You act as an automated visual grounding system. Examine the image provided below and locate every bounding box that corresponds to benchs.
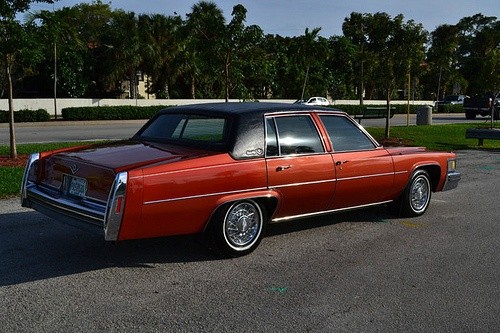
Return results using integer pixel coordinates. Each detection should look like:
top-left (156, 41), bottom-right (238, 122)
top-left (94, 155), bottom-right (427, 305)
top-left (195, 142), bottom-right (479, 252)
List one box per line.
top-left (465, 126), bottom-right (500, 147)
top-left (354, 106), bottom-right (397, 125)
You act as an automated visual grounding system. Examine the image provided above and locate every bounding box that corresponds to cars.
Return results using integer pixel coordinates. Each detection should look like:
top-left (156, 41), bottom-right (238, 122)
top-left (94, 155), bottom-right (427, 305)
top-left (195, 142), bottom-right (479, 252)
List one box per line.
top-left (293, 96), bottom-right (330, 106)
top-left (435, 94), bottom-right (471, 106)
top-left (20, 102), bottom-right (461, 257)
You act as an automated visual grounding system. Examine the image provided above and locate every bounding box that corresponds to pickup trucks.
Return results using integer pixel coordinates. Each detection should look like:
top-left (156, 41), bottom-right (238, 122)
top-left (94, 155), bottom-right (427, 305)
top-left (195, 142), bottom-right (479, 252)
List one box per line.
top-left (463, 89), bottom-right (500, 120)
